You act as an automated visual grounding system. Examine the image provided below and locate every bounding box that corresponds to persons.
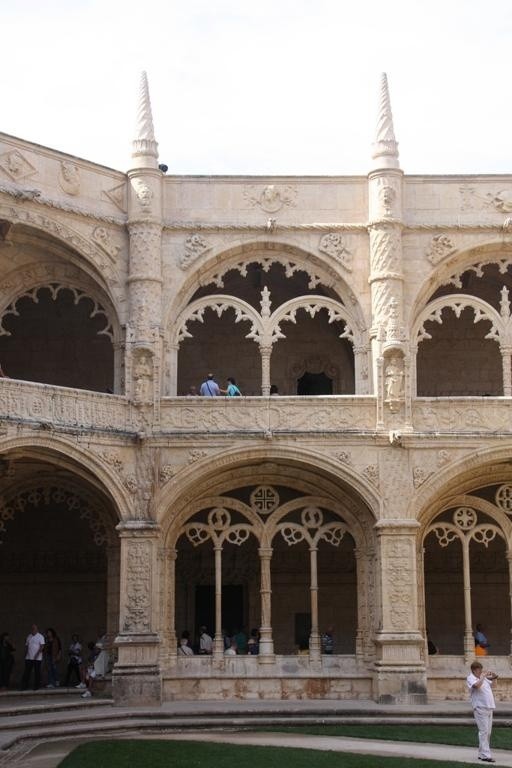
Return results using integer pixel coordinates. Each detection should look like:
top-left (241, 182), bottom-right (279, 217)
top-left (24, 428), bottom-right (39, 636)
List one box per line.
top-left (269, 384), bottom-right (280, 397)
top-left (132, 357), bottom-right (151, 400)
top-left (20, 623), bottom-right (106, 697)
top-left (468, 661), bottom-right (498, 762)
top-left (386, 357), bottom-right (405, 401)
top-left (426, 629), bottom-right (437, 655)
top-left (185, 385), bottom-right (199, 396)
top-left (297, 639), bottom-right (309, 655)
top-left (200, 373), bottom-right (221, 397)
top-left (219, 375), bottom-right (243, 398)
top-left (2, 632), bottom-right (16, 692)
top-left (177, 625), bottom-right (261, 656)
top-left (475, 624), bottom-right (490, 656)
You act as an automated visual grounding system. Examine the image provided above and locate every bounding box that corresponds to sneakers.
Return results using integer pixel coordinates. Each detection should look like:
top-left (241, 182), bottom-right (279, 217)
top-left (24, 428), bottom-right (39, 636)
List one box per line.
top-left (75, 682), bottom-right (86, 689)
top-left (82, 691), bottom-right (91, 698)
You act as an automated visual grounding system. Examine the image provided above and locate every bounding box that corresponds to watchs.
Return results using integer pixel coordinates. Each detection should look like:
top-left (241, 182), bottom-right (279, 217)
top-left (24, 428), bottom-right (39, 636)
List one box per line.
top-left (320, 625), bottom-right (335, 654)
